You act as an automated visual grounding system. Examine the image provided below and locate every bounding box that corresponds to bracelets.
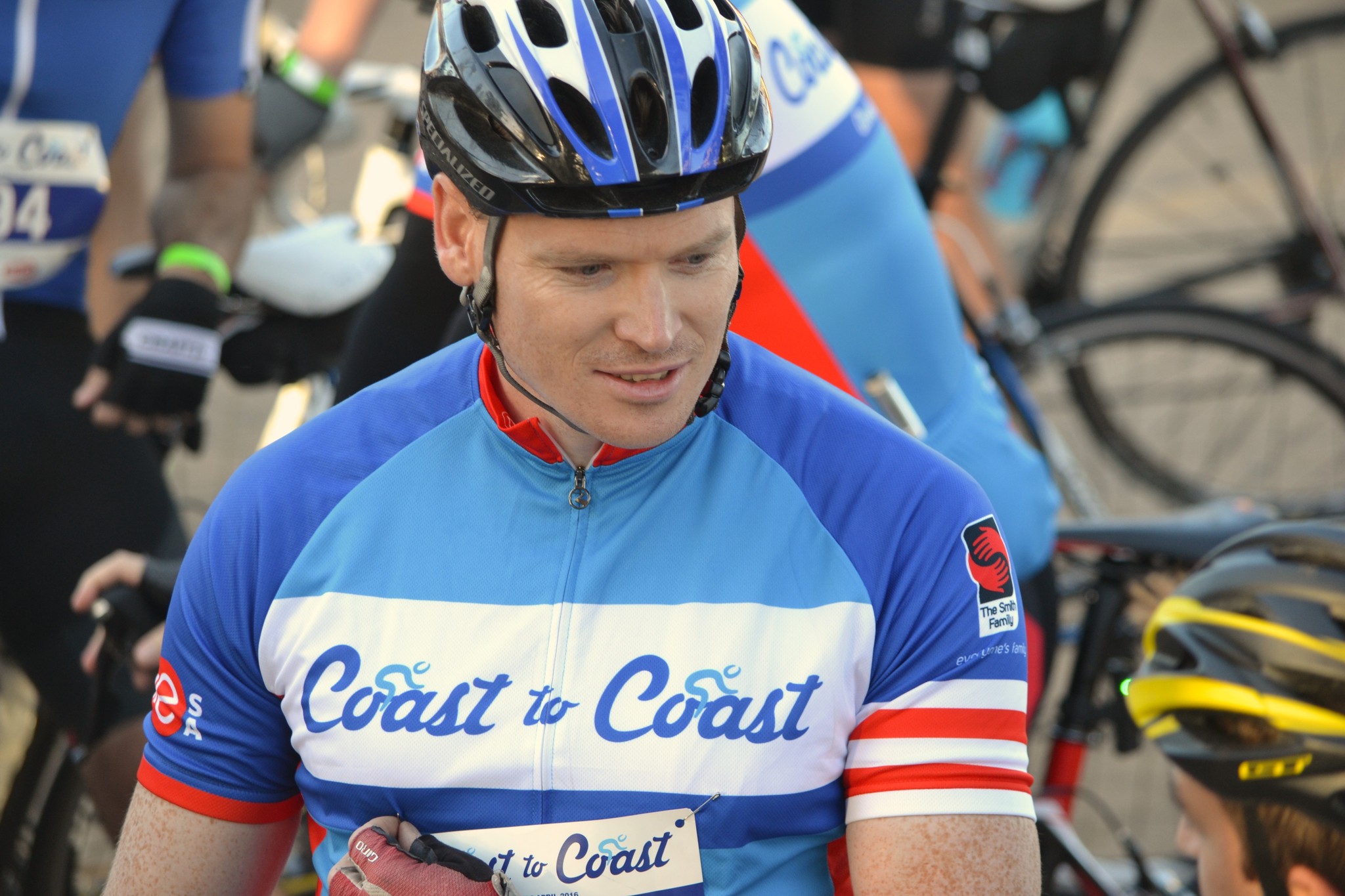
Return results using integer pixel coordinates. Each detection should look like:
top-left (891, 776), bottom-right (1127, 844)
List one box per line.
top-left (279, 47), bottom-right (335, 105)
top-left (155, 241), bottom-right (233, 293)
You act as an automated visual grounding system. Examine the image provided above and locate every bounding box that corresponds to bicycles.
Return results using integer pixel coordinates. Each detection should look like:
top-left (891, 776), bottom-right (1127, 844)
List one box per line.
top-left (841, 2), bottom-right (1345, 896)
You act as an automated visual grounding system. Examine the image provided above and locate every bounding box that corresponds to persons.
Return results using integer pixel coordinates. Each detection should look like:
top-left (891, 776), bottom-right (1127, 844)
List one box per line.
top-left (1126, 515), bottom-right (1345, 896)
top-left (102, 0), bottom-right (1041, 895)
top-left (249, 0), bottom-right (1060, 729)
top-left (2, 1), bottom-right (263, 845)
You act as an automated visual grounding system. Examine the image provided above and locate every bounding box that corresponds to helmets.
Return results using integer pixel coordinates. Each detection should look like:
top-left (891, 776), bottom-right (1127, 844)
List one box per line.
top-left (418, 0), bottom-right (774, 218)
top-left (1119, 515), bottom-right (1345, 820)
